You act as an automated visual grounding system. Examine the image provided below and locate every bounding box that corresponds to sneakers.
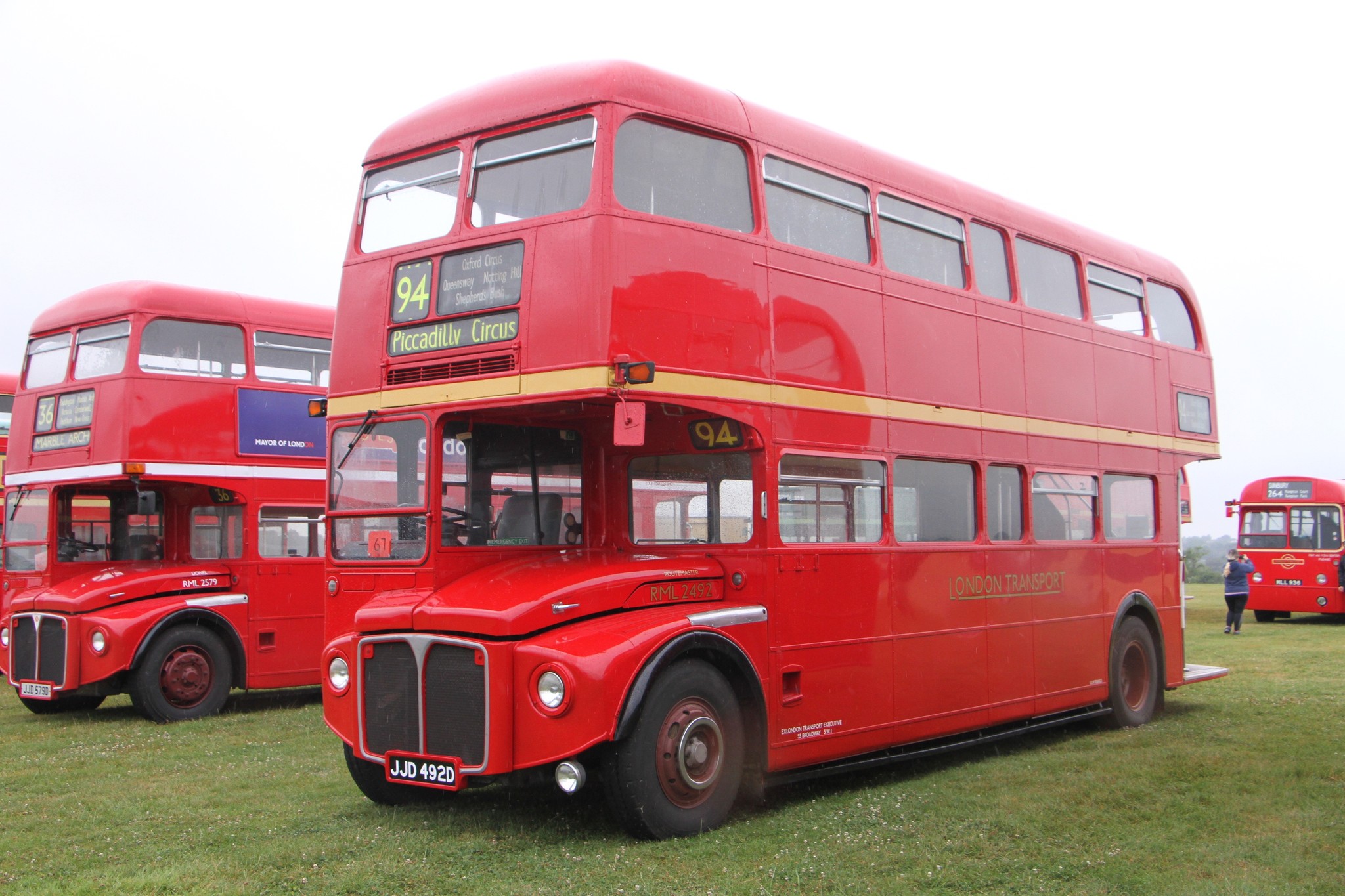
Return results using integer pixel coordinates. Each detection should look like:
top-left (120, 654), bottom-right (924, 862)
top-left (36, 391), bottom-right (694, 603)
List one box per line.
top-left (1233, 629), bottom-right (1240, 635)
top-left (1224, 626), bottom-right (1232, 633)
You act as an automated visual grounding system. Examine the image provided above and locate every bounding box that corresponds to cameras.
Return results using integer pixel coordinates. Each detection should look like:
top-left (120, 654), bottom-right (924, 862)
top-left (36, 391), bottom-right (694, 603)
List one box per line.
top-left (1239, 555), bottom-right (1245, 559)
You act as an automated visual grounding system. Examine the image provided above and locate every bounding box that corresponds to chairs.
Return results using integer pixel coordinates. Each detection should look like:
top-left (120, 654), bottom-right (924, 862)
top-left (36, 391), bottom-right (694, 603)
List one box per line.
top-left (110, 534), bottom-right (158, 560)
top-left (495, 493), bottom-right (563, 545)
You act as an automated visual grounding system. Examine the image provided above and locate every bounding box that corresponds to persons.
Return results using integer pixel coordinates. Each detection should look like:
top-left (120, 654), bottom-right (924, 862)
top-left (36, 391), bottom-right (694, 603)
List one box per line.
top-left (1309, 509), bottom-right (1341, 549)
top-left (1223, 548), bottom-right (1255, 634)
top-left (1337, 539), bottom-right (1345, 594)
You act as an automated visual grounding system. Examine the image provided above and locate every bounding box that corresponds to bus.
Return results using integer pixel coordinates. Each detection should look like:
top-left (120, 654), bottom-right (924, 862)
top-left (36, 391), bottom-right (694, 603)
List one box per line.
top-left (0, 279), bottom-right (710, 724)
top-left (1226, 476), bottom-right (1345, 621)
top-left (771, 458), bottom-right (1193, 600)
top-left (317, 58), bottom-right (1226, 842)
